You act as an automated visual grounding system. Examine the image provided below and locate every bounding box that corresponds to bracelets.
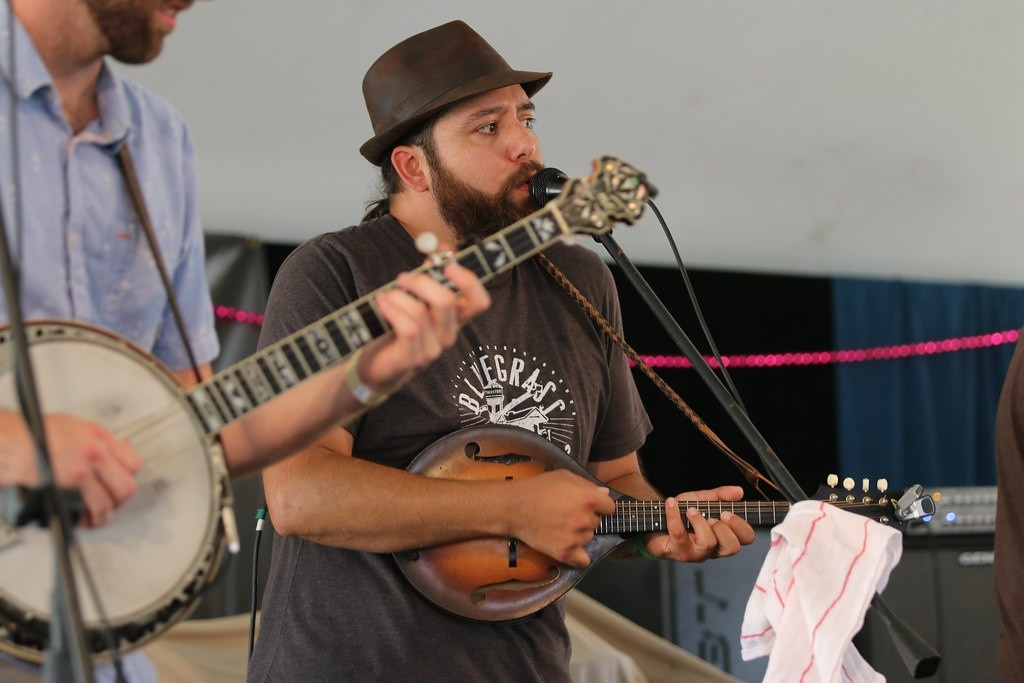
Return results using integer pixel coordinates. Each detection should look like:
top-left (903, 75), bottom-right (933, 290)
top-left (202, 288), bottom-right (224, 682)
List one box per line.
top-left (634, 534), bottom-right (662, 560)
top-left (345, 347), bottom-right (388, 407)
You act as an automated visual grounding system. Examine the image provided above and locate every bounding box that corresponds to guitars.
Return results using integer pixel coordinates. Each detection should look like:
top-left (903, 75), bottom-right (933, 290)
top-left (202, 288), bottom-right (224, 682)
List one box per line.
top-left (0, 152), bottom-right (658, 664)
top-left (390, 427), bottom-right (938, 625)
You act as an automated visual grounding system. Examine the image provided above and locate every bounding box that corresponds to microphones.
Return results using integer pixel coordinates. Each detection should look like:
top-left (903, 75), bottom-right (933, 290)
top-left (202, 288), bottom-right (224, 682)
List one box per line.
top-left (529, 167), bottom-right (573, 205)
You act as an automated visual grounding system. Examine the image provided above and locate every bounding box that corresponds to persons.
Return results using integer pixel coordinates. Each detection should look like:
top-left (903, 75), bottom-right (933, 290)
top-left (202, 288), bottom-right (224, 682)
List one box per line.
top-left (993, 327), bottom-right (1024, 683)
top-left (0, 0), bottom-right (492, 683)
top-left (245, 19), bottom-right (755, 683)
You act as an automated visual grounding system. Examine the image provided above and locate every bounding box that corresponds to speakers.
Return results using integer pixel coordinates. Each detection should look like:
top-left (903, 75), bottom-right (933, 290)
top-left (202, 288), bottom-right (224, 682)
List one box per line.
top-left (571, 532), bottom-right (1002, 683)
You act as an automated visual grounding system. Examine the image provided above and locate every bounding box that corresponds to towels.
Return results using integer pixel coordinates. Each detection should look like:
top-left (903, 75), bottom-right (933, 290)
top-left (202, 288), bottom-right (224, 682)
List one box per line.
top-left (739, 499), bottom-right (904, 683)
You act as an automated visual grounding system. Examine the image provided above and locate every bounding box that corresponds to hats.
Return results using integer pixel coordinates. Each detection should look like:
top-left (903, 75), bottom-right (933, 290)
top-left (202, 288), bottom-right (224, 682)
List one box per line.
top-left (359, 20), bottom-right (552, 163)
